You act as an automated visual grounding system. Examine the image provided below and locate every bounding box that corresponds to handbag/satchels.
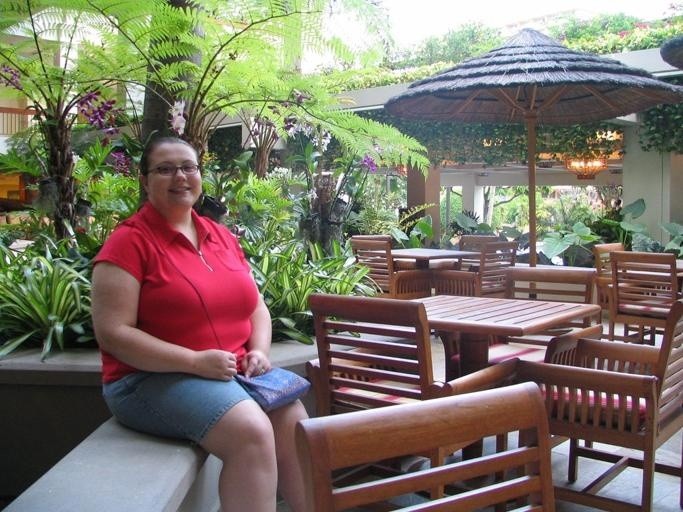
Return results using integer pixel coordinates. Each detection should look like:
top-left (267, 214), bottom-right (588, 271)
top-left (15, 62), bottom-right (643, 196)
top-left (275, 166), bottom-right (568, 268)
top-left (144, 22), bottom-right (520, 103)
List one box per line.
top-left (236, 365), bottom-right (312, 414)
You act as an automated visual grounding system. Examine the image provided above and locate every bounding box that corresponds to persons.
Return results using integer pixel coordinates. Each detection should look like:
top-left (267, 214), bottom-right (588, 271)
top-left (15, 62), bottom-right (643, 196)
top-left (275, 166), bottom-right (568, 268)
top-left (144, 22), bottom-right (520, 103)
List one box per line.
top-left (89, 136), bottom-right (310, 512)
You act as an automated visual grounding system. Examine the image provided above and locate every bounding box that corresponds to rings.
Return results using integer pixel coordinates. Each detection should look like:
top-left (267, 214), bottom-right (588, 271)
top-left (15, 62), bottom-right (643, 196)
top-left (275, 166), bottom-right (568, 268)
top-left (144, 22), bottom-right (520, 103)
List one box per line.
top-left (261, 369), bottom-right (265, 374)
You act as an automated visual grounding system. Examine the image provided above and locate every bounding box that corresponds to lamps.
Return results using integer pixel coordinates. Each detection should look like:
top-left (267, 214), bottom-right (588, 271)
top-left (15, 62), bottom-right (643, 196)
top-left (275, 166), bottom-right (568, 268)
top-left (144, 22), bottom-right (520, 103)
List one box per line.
top-left (565, 152), bottom-right (609, 179)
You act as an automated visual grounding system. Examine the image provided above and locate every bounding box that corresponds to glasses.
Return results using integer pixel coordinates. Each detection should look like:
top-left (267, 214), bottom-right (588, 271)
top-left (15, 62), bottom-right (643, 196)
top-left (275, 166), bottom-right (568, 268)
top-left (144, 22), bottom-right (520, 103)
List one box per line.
top-left (144, 164), bottom-right (201, 177)
